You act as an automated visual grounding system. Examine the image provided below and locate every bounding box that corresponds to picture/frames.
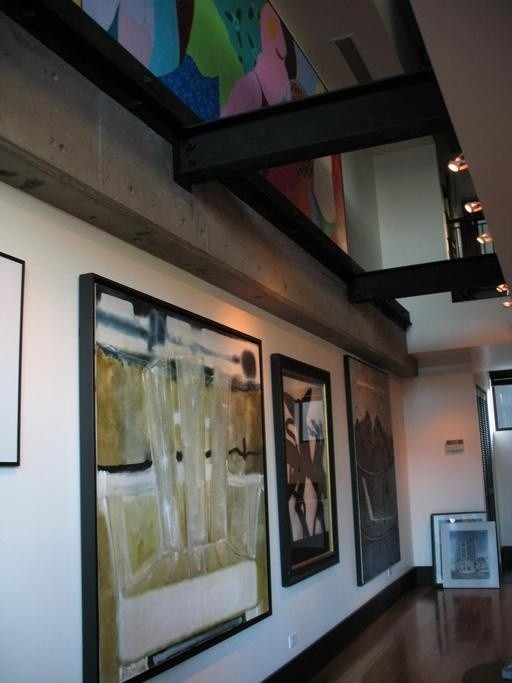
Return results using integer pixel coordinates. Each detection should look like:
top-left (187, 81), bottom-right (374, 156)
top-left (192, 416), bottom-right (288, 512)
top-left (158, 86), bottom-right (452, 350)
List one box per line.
top-left (429, 511), bottom-right (502, 591)
